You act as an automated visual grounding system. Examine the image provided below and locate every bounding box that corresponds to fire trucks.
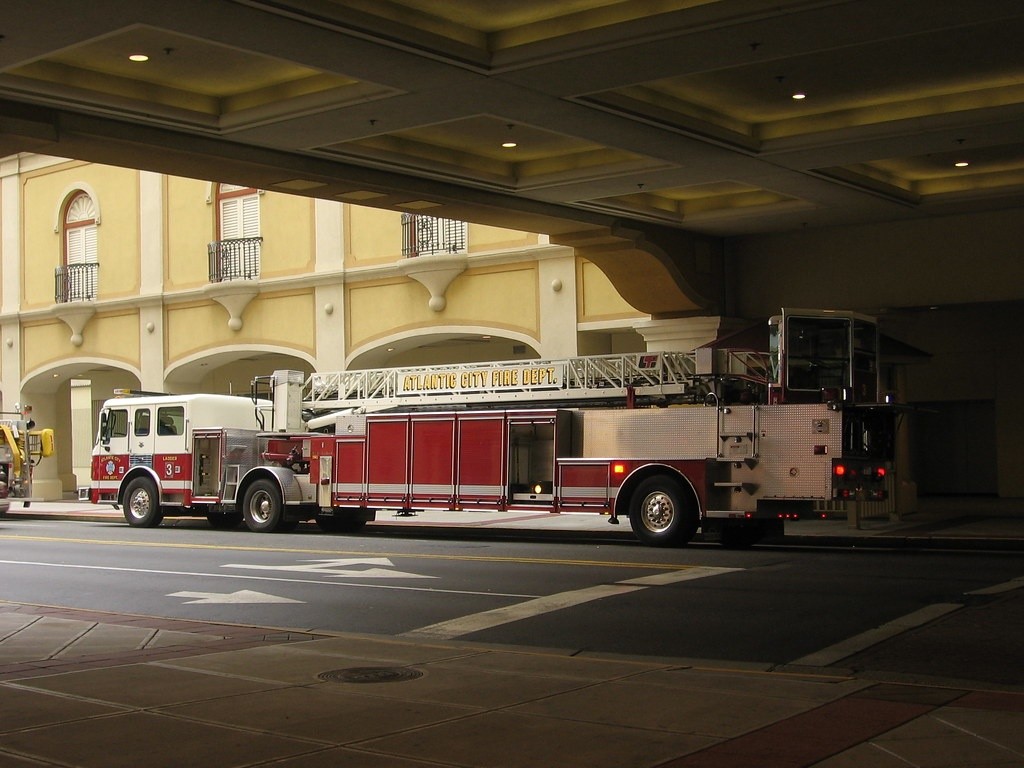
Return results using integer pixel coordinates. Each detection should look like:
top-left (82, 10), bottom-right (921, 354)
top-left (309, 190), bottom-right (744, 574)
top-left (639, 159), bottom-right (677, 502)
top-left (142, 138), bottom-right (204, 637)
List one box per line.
top-left (85, 341), bottom-right (895, 550)
top-left (0, 402), bottom-right (54, 516)
